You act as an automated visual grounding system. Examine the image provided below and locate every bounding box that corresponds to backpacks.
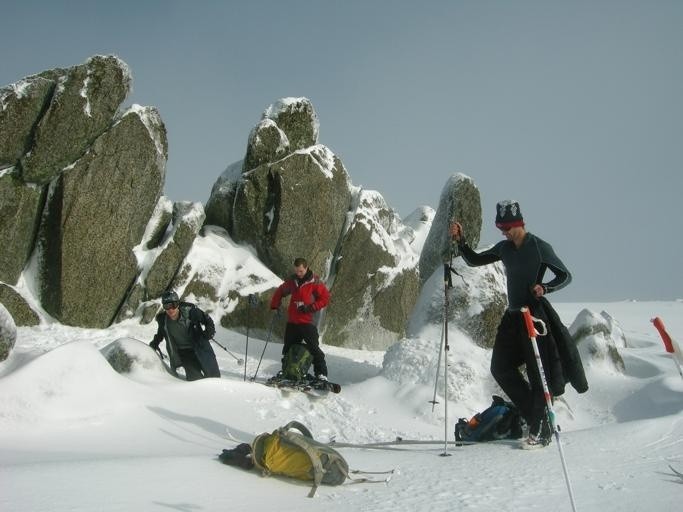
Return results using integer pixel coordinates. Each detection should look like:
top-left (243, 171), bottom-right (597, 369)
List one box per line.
top-left (251, 421), bottom-right (349, 498)
top-left (281, 343), bottom-right (313, 380)
top-left (455, 396), bottom-right (522, 446)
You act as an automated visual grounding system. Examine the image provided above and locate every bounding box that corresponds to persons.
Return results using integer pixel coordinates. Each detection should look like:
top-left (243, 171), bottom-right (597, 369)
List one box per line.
top-left (269, 258), bottom-right (331, 387)
top-left (149, 290), bottom-right (216, 380)
top-left (451, 200), bottom-right (573, 450)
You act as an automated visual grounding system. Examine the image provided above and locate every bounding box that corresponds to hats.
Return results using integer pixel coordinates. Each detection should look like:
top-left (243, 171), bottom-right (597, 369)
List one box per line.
top-left (496, 200), bottom-right (523, 227)
top-left (162, 292), bottom-right (178, 302)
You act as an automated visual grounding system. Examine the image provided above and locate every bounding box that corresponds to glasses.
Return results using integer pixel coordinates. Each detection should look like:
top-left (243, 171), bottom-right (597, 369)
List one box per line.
top-left (163, 302), bottom-right (180, 309)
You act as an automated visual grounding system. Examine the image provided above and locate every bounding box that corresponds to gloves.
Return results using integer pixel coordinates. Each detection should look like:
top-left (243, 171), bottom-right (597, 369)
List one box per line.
top-left (298, 305), bottom-right (311, 312)
